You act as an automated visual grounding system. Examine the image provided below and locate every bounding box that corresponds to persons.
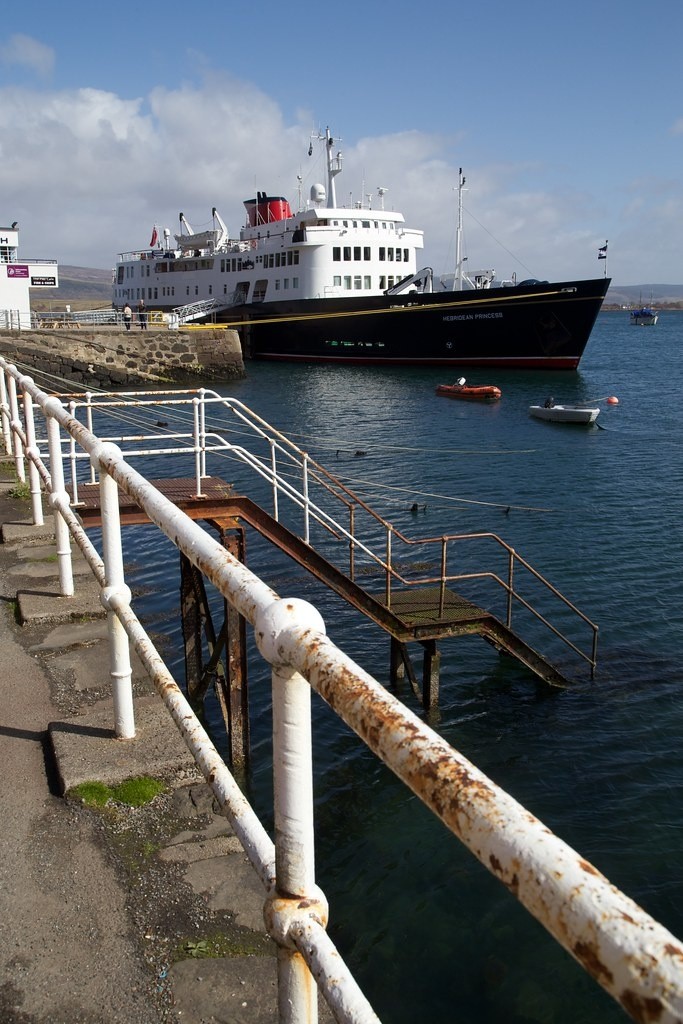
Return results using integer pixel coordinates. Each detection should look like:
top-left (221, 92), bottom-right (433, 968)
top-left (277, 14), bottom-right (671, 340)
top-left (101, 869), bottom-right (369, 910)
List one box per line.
top-left (123, 303), bottom-right (132, 331)
top-left (135, 300), bottom-right (148, 330)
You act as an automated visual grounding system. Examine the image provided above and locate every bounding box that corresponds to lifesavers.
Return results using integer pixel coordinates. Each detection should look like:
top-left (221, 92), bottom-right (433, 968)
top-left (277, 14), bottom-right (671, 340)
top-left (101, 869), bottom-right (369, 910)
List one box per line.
top-left (251, 240), bottom-right (257, 248)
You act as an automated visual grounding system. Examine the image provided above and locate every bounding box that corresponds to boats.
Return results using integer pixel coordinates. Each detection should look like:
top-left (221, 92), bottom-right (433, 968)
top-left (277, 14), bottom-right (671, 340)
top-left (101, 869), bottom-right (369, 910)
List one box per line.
top-left (435, 377), bottom-right (501, 400)
top-left (528, 394), bottom-right (600, 423)
top-left (111, 126), bottom-right (614, 373)
top-left (630, 306), bottom-right (659, 325)
top-left (171, 231), bottom-right (223, 251)
